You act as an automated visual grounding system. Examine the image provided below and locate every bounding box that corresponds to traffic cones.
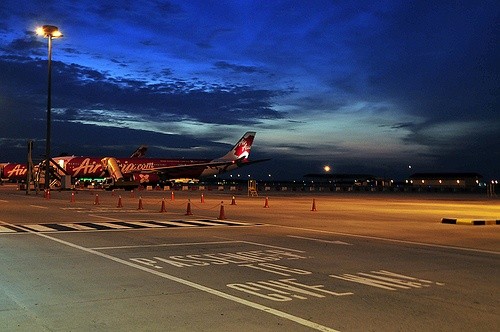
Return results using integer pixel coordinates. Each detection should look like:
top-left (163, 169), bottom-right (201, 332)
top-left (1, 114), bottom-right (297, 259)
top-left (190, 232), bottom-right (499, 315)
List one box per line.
top-left (216, 201), bottom-right (228, 220)
top-left (199, 193), bottom-right (206, 204)
top-left (311, 199), bottom-right (317, 211)
top-left (184, 199), bottom-right (193, 216)
top-left (230, 195), bottom-right (237, 205)
top-left (117, 196), bottom-right (123, 208)
top-left (94, 194), bottom-right (100, 205)
top-left (137, 196), bottom-right (144, 210)
top-left (264, 197), bottom-right (270, 208)
top-left (170, 191), bottom-right (175, 201)
top-left (111, 189), bottom-right (114, 198)
top-left (70, 192), bottom-right (76, 203)
top-left (160, 197), bottom-right (167, 213)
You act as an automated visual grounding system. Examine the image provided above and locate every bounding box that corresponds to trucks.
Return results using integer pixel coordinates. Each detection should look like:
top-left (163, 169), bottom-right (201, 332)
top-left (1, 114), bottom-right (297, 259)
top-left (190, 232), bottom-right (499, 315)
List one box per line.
top-left (102, 177), bottom-right (139, 191)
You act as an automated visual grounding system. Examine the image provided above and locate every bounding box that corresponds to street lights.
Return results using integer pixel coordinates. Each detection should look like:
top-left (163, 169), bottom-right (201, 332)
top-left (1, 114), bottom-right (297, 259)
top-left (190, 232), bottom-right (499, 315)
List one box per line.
top-left (33, 24), bottom-right (63, 192)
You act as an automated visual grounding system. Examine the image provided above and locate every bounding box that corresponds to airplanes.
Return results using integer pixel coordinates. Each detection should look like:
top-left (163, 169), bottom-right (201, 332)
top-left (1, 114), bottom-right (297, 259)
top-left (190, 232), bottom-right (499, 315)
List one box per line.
top-left (0, 163), bottom-right (39, 178)
top-left (38, 131), bottom-right (271, 179)
